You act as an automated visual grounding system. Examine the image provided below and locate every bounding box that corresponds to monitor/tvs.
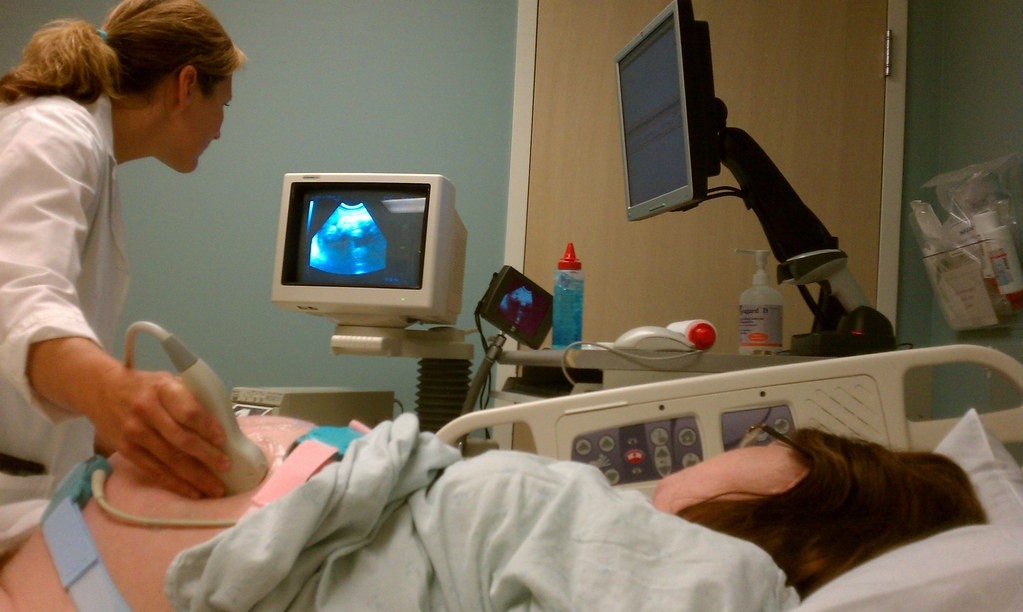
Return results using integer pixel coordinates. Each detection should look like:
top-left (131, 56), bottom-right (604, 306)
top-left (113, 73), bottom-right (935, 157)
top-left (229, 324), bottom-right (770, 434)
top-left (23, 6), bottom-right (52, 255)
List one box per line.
top-left (268, 171), bottom-right (467, 328)
top-left (611, 0), bottom-right (894, 361)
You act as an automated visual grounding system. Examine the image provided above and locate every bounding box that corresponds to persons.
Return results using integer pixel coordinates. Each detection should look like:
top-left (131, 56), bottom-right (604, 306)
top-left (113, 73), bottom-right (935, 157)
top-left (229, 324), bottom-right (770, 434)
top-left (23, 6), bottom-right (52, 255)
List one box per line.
top-left (0, 416), bottom-right (991, 612)
top-left (0, 1), bottom-right (247, 503)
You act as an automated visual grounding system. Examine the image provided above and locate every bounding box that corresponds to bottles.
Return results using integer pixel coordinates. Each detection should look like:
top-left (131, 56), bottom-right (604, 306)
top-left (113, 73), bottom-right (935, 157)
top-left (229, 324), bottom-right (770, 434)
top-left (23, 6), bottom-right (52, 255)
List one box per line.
top-left (973, 210), bottom-right (1023, 308)
top-left (552, 243), bottom-right (583, 350)
top-left (666, 319), bottom-right (717, 350)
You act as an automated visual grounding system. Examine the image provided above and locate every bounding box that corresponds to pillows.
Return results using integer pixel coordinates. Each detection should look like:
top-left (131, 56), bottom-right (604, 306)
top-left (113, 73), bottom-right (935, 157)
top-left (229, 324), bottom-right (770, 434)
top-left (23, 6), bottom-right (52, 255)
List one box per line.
top-left (772, 407), bottom-right (1022, 611)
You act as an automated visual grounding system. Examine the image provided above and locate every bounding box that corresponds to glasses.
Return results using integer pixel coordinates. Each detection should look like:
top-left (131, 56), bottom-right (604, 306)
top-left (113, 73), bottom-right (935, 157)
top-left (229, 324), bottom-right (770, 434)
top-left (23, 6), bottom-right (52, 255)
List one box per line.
top-left (740, 423), bottom-right (811, 458)
